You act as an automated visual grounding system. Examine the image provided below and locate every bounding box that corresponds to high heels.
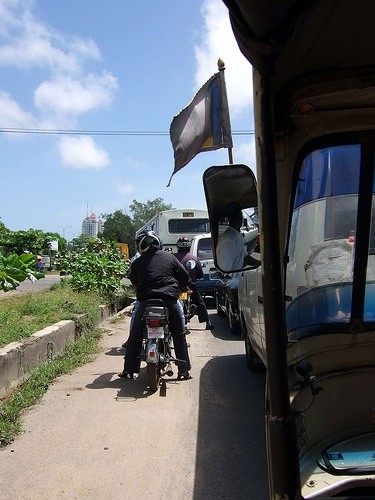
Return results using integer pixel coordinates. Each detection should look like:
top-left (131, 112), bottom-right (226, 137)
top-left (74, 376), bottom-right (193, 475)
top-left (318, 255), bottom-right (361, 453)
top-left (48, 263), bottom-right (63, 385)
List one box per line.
top-left (118, 370), bottom-right (134, 379)
top-left (178, 372), bottom-right (193, 379)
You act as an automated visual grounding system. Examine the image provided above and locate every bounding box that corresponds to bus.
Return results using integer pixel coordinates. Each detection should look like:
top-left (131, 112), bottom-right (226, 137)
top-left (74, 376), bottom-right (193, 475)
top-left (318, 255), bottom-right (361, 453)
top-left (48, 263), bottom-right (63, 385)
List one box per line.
top-left (135, 209), bottom-right (211, 256)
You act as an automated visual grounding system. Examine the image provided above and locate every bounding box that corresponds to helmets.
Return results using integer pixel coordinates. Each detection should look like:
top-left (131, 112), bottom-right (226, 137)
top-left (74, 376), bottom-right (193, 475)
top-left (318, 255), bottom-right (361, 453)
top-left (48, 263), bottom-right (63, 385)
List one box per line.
top-left (176, 237), bottom-right (191, 252)
top-left (135, 229), bottom-right (161, 255)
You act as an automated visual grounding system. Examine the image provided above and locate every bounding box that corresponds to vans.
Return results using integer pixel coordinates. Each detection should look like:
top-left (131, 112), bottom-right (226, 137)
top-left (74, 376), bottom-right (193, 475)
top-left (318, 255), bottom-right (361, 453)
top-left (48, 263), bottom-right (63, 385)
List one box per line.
top-left (237, 147), bottom-right (375, 371)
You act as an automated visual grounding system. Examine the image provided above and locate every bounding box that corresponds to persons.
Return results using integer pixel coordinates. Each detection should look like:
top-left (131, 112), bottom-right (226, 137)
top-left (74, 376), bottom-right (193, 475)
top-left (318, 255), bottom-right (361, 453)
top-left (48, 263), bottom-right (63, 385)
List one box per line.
top-left (172, 236), bottom-right (215, 330)
top-left (118, 230), bottom-right (192, 379)
top-left (36, 259), bottom-right (43, 272)
top-left (216, 203), bottom-right (260, 271)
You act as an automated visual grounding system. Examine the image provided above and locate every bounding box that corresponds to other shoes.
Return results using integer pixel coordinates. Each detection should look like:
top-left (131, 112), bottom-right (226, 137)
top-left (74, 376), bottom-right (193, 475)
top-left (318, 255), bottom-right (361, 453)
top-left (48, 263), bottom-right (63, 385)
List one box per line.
top-left (186, 343), bottom-right (190, 348)
top-left (122, 339), bottom-right (129, 347)
top-left (206, 325), bottom-right (215, 330)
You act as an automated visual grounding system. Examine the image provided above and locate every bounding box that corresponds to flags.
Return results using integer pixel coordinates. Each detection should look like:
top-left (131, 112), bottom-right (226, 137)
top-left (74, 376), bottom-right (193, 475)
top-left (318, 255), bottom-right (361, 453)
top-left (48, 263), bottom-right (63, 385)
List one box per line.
top-left (167, 73), bottom-right (234, 187)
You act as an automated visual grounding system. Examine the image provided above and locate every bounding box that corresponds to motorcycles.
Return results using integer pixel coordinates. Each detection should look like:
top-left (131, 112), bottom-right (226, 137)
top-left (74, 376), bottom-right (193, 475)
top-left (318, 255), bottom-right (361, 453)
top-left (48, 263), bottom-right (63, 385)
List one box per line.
top-left (139, 259), bottom-right (208, 393)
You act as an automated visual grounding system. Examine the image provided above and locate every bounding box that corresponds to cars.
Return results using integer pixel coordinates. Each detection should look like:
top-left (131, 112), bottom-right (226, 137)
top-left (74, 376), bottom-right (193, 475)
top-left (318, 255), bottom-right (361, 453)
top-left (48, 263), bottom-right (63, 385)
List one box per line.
top-left (187, 207), bottom-right (259, 338)
